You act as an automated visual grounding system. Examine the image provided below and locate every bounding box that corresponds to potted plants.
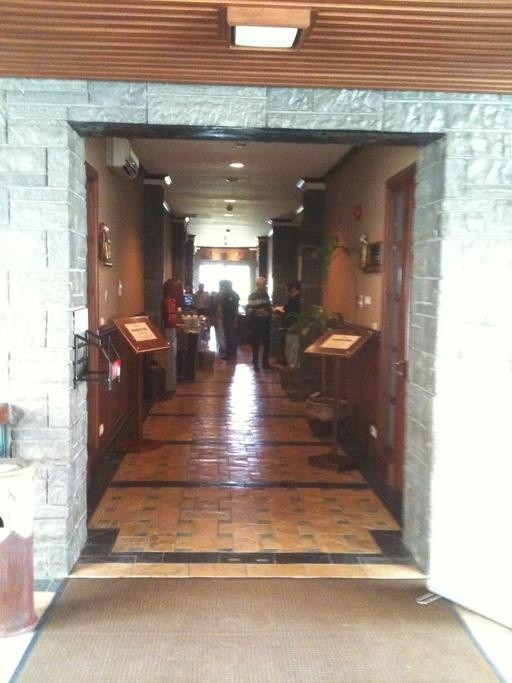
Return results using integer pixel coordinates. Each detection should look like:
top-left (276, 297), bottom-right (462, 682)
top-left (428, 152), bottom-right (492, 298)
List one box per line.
top-left (276, 232), bottom-right (352, 438)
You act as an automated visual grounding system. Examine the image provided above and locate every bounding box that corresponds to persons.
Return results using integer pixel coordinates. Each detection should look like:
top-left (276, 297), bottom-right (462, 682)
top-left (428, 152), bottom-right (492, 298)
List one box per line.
top-left (186, 280), bottom-right (240, 361)
top-left (247, 277), bottom-right (275, 372)
top-left (272, 281), bottom-right (300, 370)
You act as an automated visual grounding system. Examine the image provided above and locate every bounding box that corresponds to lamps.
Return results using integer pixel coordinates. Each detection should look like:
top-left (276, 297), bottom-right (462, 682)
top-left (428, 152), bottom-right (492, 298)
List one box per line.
top-left (226, 5), bottom-right (315, 54)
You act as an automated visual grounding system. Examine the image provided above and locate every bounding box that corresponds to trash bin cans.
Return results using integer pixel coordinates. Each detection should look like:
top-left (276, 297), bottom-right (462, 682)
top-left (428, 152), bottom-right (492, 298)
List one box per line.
top-left (1, 458), bottom-right (39, 637)
top-left (145, 360), bottom-right (166, 400)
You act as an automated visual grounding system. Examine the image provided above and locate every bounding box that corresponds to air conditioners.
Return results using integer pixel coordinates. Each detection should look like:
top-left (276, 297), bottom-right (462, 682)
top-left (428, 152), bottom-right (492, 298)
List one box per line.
top-left (105, 135), bottom-right (140, 180)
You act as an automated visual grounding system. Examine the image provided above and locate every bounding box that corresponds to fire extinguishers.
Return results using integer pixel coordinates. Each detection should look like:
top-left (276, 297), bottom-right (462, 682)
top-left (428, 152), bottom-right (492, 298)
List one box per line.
top-left (163, 295), bottom-right (176, 326)
